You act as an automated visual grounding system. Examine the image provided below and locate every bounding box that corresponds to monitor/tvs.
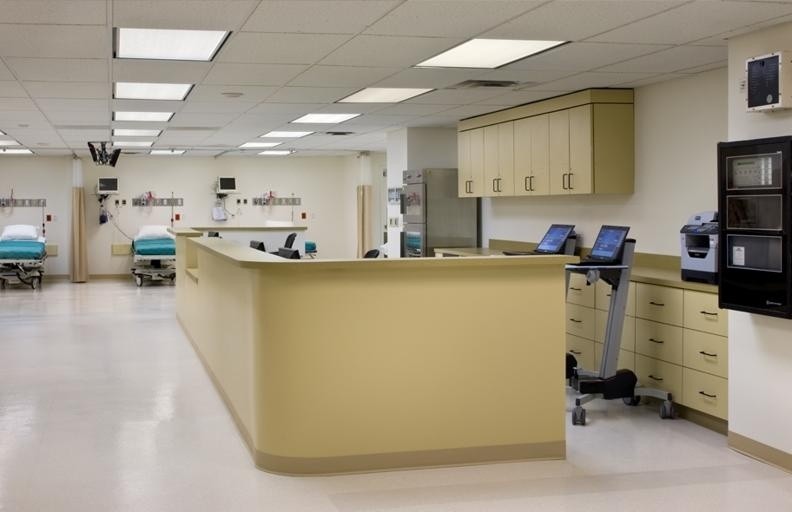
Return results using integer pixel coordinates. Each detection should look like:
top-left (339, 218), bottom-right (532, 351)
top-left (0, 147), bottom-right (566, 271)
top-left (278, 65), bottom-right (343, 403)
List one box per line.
top-left (278, 247), bottom-right (300, 259)
top-left (218, 175), bottom-right (237, 191)
top-left (98, 178), bottom-right (118, 195)
top-left (249, 240), bottom-right (266, 251)
top-left (208, 231), bottom-right (219, 236)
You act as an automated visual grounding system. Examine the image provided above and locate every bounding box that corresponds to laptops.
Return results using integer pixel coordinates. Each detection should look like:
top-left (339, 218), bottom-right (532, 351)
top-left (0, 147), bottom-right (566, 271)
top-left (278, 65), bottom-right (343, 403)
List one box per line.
top-left (581, 224), bottom-right (630, 264)
top-left (503, 223), bottom-right (576, 256)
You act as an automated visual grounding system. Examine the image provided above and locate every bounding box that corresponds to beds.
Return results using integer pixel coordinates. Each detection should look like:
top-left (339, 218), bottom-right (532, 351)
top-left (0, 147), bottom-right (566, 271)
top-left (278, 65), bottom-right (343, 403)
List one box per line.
top-left (132, 235), bottom-right (177, 287)
top-left (0, 238), bottom-right (48, 289)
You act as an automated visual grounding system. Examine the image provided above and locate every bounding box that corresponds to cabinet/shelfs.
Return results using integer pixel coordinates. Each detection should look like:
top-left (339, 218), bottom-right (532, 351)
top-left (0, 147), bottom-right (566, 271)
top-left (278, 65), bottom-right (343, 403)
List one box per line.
top-left (514, 99), bottom-right (550, 197)
top-left (484, 106), bottom-right (513, 196)
top-left (550, 88), bottom-right (635, 196)
top-left (715, 136), bottom-right (792, 319)
top-left (458, 116), bottom-right (485, 197)
top-left (567, 267), bottom-right (728, 435)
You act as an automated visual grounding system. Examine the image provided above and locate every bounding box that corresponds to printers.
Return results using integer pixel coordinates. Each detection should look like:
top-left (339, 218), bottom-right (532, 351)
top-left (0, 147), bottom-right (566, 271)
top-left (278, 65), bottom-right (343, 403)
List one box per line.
top-left (679, 209), bottom-right (718, 285)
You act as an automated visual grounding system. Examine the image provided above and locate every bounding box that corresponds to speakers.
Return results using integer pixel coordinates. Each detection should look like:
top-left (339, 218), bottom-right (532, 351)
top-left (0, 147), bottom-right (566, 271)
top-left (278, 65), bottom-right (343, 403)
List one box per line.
top-left (743, 49), bottom-right (791, 113)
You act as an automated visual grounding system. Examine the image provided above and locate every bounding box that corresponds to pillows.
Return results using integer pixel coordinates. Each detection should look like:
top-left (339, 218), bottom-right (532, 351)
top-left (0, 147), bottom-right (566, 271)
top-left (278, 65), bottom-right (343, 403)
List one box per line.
top-left (1, 225), bottom-right (38, 241)
top-left (139, 225), bottom-right (173, 240)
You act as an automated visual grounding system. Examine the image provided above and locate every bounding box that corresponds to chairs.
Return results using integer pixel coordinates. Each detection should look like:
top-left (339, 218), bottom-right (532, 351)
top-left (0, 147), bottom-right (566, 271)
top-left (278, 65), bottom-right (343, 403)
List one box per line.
top-left (270, 233), bottom-right (297, 255)
